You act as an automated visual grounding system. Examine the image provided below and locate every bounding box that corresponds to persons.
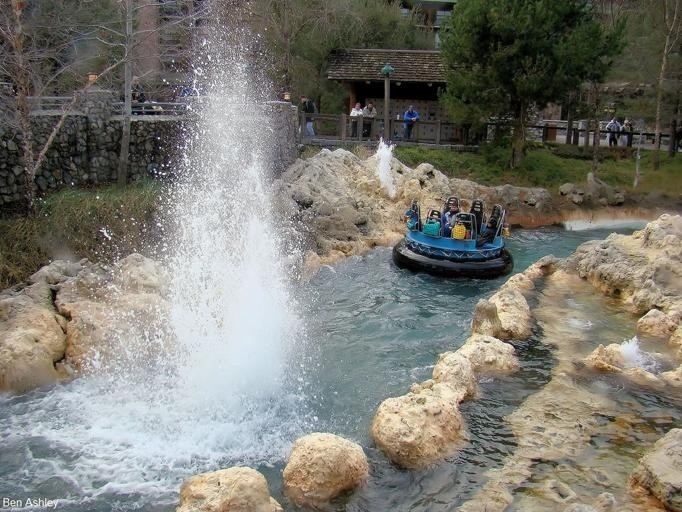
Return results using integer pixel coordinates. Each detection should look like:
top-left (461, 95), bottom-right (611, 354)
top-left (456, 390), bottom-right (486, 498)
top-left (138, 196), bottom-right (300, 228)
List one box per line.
top-left (304, 98), bottom-right (317, 136)
top-left (444, 200), bottom-right (460, 228)
top-left (119, 72), bottom-right (146, 115)
top-left (618, 119), bottom-right (628, 147)
top-left (474, 218), bottom-right (496, 248)
top-left (360, 103), bottom-right (377, 138)
top-left (606, 117), bottom-right (620, 147)
top-left (626, 122), bottom-right (634, 148)
top-left (403, 105), bottom-right (419, 141)
top-left (441, 214), bottom-right (452, 238)
top-left (349, 102), bottom-right (363, 138)
top-left (297, 96), bottom-right (308, 135)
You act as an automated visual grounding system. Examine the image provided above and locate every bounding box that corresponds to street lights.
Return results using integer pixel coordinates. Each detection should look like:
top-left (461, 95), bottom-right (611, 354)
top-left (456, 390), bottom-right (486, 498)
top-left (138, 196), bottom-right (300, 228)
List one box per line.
top-left (378, 63), bottom-right (395, 141)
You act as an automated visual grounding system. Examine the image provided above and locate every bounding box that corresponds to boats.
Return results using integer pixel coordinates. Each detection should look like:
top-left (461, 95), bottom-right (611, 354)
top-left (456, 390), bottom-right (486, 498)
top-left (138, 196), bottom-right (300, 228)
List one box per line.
top-left (392, 198), bottom-right (515, 279)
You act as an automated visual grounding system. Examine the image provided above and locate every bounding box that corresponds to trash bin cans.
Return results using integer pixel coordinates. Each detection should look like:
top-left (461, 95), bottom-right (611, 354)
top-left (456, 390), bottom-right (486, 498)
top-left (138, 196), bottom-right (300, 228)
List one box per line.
top-left (543, 123), bottom-right (556, 141)
